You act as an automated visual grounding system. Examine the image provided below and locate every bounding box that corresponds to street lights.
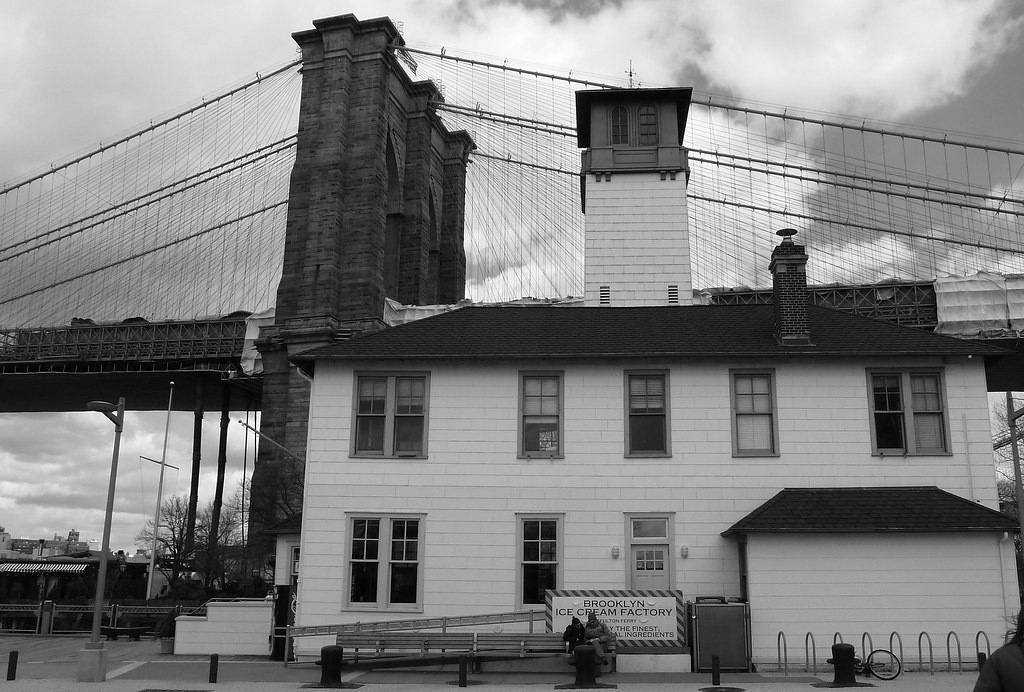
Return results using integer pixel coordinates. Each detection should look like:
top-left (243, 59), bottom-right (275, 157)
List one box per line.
top-left (75, 396), bottom-right (126, 684)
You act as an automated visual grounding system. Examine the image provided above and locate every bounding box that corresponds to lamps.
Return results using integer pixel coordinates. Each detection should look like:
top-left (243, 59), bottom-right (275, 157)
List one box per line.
top-left (612, 546), bottom-right (619, 558)
top-left (681, 544), bottom-right (688, 558)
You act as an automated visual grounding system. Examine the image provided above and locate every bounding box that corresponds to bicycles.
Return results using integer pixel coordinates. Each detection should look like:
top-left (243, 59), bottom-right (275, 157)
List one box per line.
top-left (826, 649), bottom-right (902, 681)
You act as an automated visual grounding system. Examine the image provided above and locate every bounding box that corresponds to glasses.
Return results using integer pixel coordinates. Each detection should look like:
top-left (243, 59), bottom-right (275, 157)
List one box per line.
top-left (590, 621), bottom-right (595, 623)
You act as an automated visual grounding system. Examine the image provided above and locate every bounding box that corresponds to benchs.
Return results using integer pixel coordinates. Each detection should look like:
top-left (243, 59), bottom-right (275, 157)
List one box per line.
top-left (99, 626), bottom-right (150, 642)
top-left (336, 632), bottom-right (616, 687)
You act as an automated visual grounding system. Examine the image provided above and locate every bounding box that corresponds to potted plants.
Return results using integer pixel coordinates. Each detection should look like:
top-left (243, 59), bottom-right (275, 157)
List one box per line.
top-left (152, 611), bottom-right (179, 653)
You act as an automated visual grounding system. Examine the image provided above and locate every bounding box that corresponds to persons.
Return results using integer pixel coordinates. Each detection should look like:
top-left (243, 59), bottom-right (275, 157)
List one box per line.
top-left (972, 600), bottom-right (1024, 692)
top-left (562, 612), bottom-right (611, 678)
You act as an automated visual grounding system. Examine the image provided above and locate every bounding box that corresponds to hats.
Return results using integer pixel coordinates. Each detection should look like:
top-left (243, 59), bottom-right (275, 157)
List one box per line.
top-left (588, 613), bottom-right (595, 620)
top-left (572, 616), bottom-right (580, 627)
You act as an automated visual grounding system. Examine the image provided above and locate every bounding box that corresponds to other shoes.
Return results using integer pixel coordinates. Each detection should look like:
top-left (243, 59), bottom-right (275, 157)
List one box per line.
top-left (595, 671), bottom-right (601, 676)
top-left (602, 656), bottom-right (606, 661)
